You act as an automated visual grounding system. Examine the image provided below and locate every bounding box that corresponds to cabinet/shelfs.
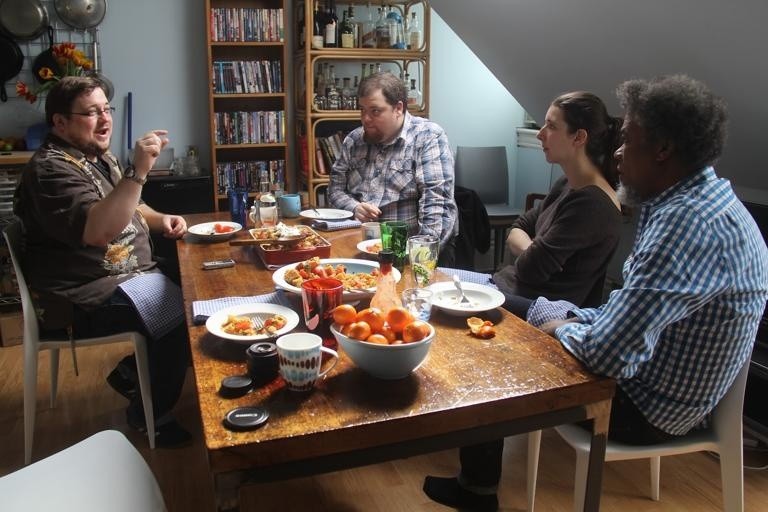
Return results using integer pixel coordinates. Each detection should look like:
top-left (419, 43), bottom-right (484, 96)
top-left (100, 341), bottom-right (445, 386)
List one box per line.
top-left (141, 176), bottom-right (214, 272)
top-left (516, 125), bottom-right (569, 212)
top-left (205, 0), bottom-right (292, 213)
top-left (291, 1), bottom-right (432, 212)
top-left (736, 185), bottom-right (766, 512)
top-left (0, 148), bottom-right (39, 316)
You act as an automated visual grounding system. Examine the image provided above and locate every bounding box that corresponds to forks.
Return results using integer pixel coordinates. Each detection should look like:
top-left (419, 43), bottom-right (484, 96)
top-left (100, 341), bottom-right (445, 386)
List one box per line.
top-left (252, 316), bottom-right (277, 343)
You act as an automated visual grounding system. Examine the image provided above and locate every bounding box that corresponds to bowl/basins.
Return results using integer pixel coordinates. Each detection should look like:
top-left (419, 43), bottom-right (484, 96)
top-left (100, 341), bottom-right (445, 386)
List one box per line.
top-left (329, 310), bottom-right (435, 382)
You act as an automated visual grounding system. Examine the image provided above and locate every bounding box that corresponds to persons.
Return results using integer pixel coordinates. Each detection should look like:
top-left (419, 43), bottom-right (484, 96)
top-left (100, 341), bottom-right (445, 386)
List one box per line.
top-left (322, 71), bottom-right (468, 270)
top-left (429, 88), bottom-right (624, 311)
top-left (420, 72), bottom-right (768, 511)
top-left (9, 71), bottom-right (196, 452)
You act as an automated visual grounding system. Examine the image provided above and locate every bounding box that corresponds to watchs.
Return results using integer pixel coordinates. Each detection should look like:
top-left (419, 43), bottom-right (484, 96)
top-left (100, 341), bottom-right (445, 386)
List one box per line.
top-left (122, 164), bottom-right (147, 187)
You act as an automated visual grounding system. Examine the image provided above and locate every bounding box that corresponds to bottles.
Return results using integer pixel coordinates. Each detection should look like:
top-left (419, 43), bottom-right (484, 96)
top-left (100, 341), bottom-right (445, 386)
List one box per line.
top-left (314, 61), bottom-right (423, 110)
top-left (313, 0), bottom-right (423, 51)
top-left (245, 341), bottom-right (281, 378)
top-left (254, 170), bottom-right (278, 226)
top-left (369, 251), bottom-right (403, 309)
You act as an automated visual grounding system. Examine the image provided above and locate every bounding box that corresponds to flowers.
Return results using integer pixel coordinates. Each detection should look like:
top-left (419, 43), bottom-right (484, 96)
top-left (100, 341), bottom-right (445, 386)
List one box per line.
top-left (14, 39), bottom-right (94, 111)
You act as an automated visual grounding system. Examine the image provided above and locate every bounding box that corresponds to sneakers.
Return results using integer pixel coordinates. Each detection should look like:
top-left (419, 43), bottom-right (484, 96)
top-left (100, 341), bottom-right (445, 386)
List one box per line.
top-left (125, 398), bottom-right (193, 450)
top-left (106, 353), bottom-right (150, 403)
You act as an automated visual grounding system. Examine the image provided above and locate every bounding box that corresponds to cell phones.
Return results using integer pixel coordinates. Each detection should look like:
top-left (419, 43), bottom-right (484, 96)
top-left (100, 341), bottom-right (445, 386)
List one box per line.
top-left (203, 259), bottom-right (235, 270)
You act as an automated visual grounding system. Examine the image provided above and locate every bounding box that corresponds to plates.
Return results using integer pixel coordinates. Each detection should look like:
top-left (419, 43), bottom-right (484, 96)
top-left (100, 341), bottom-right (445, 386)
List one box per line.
top-left (355, 238), bottom-right (394, 257)
top-left (298, 208), bottom-right (355, 223)
top-left (187, 221), bottom-right (243, 244)
top-left (419, 282), bottom-right (506, 318)
top-left (205, 302), bottom-right (299, 346)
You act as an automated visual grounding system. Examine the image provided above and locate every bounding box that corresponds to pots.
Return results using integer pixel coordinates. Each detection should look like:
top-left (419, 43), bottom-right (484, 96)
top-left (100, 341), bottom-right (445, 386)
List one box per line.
top-left (1, 1), bottom-right (115, 104)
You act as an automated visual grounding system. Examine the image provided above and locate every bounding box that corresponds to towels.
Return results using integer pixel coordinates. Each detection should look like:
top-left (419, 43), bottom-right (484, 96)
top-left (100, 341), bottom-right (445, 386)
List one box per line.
top-left (526, 295), bottom-right (581, 330)
top-left (434, 264), bottom-right (498, 289)
top-left (308, 218), bottom-right (362, 232)
top-left (118, 270), bottom-right (188, 343)
top-left (192, 288), bottom-right (298, 327)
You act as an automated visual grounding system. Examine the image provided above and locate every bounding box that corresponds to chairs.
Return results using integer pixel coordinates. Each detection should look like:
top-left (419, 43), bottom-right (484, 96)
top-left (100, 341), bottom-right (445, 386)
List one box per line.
top-left (523, 343), bottom-right (754, 512)
top-left (523, 192), bottom-right (548, 212)
top-left (452, 144), bottom-right (520, 276)
top-left (1, 222), bottom-right (161, 467)
top-left (438, 179), bottom-right (481, 272)
top-left (0, 429), bottom-right (173, 512)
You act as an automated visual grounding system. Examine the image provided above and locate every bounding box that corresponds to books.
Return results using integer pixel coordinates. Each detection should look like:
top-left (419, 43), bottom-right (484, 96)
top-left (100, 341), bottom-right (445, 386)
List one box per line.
top-left (209, 6), bottom-right (288, 196)
top-left (299, 127), bottom-right (350, 178)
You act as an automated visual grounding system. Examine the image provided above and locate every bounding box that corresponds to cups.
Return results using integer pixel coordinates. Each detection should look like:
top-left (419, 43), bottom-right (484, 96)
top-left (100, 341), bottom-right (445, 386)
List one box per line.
top-left (408, 234), bottom-right (438, 289)
top-left (227, 189), bottom-right (248, 227)
top-left (401, 288), bottom-right (434, 324)
top-left (299, 278), bottom-right (343, 337)
top-left (378, 220), bottom-right (408, 273)
top-left (279, 191), bottom-right (301, 219)
top-left (275, 332), bottom-right (339, 393)
top-left (361, 221), bottom-right (380, 241)
top-left (175, 157), bottom-right (202, 178)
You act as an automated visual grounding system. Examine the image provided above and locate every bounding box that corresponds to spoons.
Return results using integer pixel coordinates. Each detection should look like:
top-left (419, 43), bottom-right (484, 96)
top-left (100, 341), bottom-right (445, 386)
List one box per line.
top-left (451, 273), bottom-right (475, 310)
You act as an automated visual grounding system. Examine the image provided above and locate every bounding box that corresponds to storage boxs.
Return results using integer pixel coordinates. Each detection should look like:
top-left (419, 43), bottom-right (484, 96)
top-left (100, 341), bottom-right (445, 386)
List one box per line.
top-left (1, 313), bottom-right (24, 348)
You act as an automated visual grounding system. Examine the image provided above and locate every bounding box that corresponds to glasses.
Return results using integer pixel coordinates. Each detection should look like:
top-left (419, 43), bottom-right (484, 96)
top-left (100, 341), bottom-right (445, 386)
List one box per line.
top-left (55, 106), bottom-right (117, 117)
